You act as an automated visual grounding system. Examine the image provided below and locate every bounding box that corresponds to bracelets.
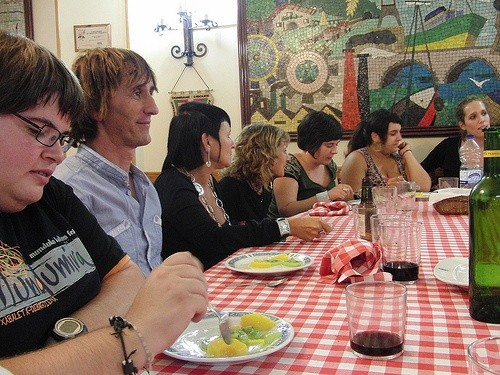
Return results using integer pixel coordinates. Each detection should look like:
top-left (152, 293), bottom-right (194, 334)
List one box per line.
top-left (108, 315), bottom-right (140, 375)
top-left (402, 149), bottom-right (413, 157)
top-left (316, 191), bottom-right (332, 204)
top-left (122, 318), bottom-right (154, 373)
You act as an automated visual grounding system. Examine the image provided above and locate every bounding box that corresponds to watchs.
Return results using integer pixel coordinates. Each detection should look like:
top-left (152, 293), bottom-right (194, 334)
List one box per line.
top-left (52, 317), bottom-right (88, 343)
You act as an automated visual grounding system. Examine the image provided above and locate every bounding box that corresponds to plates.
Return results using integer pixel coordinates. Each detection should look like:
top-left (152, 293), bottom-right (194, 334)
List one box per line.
top-left (162, 311), bottom-right (294, 367)
top-left (347, 199), bottom-right (362, 205)
top-left (416, 191), bottom-right (431, 201)
top-left (226, 251), bottom-right (314, 277)
top-left (434, 257), bottom-right (469, 291)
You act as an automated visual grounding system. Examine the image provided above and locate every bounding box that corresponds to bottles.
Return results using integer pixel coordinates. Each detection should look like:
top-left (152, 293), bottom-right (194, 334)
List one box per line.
top-left (356, 177), bottom-right (376, 241)
top-left (467, 127), bottom-right (500, 324)
top-left (458, 133), bottom-right (483, 189)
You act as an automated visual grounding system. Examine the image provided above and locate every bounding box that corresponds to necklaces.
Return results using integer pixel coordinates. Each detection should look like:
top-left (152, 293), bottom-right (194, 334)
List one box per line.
top-left (182, 166), bottom-right (233, 228)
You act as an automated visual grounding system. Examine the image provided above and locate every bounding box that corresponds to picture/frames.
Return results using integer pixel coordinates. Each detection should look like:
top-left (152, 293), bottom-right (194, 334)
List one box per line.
top-left (72, 23), bottom-right (112, 53)
top-left (0, 1), bottom-right (36, 40)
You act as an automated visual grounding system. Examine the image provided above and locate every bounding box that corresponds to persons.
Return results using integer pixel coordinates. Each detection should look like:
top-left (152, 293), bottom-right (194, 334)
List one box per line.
top-left (419, 95), bottom-right (491, 192)
top-left (271, 109), bottom-right (356, 219)
top-left (153, 102), bottom-right (332, 279)
top-left (216, 122), bottom-right (292, 226)
top-left (0, 25), bottom-right (209, 375)
top-left (49, 45), bottom-right (164, 280)
top-left (340, 107), bottom-right (432, 199)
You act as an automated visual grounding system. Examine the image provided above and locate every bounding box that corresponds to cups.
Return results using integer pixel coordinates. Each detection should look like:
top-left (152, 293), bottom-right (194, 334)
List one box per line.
top-left (378, 220), bottom-right (423, 283)
top-left (352, 205), bottom-right (360, 239)
top-left (374, 178), bottom-right (418, 262)
top-left (438, 177), bottom-right (459, 188)
top-left (466, 336), bottom-right (500, 375)
top-left (344, 280), bottom-right (408, 360)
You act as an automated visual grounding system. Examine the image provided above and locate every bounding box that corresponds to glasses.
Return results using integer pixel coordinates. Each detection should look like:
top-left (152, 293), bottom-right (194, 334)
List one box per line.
top-left (10, 111), bottom-right (76, 153)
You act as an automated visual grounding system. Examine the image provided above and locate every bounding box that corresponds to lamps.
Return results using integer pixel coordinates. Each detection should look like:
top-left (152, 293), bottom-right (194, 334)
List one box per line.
top-left (152, 1), bottom-right (216, 59)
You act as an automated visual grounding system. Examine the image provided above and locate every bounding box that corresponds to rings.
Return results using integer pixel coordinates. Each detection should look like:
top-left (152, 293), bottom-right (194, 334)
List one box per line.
top-left (318, 229), bottom-right (324, 234)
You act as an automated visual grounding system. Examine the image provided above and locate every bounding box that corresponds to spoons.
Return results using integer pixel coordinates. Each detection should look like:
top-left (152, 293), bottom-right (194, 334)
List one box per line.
top-left (207, 300), bottom-right (233, 345)
top-left (225, 277), bottom-right (289, 287)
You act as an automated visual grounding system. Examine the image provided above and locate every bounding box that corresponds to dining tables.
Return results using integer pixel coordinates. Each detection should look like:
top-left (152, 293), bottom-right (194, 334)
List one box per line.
top-left (133, 193), bottom-right (500, 375)
